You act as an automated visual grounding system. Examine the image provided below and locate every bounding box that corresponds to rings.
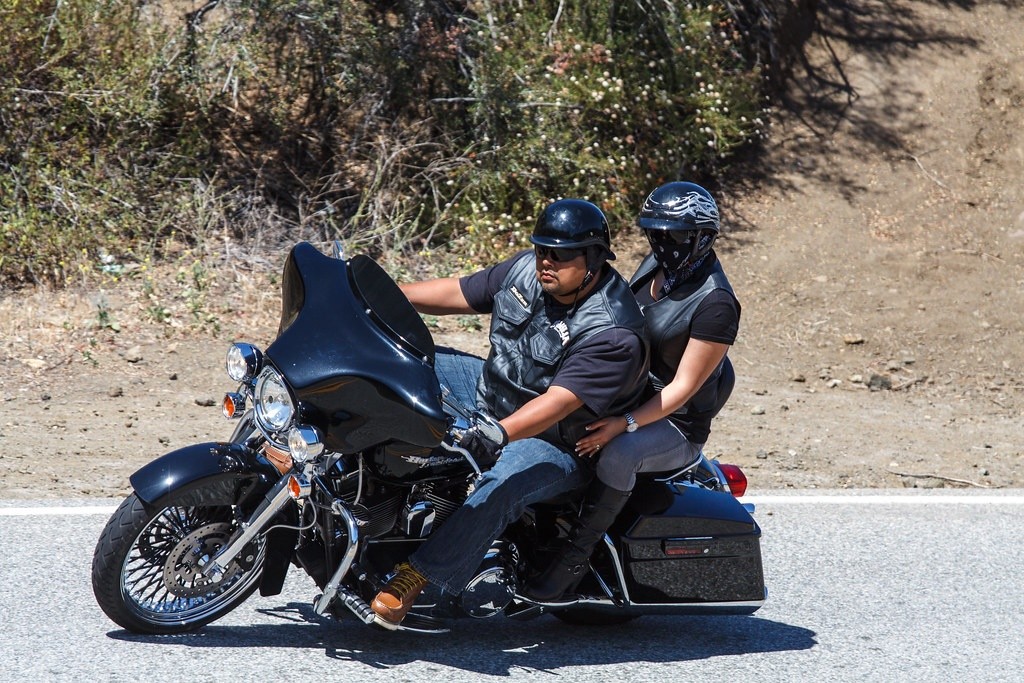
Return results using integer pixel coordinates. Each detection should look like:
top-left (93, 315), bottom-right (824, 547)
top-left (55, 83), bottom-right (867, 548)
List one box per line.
top-left (597, 444), bottom-right (600, 451)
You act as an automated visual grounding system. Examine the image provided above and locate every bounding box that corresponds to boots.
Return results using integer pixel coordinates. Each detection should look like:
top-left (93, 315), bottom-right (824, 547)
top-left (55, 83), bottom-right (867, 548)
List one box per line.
top-left (523, 473), bottom-right (633, 602)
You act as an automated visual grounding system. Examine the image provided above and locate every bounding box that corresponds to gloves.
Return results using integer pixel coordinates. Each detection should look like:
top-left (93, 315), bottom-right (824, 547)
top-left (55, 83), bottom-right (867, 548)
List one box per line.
top-left (459, 418), bottom-right (509, 470)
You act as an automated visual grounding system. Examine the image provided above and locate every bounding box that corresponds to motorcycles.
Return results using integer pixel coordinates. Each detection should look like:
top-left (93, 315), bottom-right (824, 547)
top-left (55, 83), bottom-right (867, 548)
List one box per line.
top-left (88, 240), bottom-right (771, 636)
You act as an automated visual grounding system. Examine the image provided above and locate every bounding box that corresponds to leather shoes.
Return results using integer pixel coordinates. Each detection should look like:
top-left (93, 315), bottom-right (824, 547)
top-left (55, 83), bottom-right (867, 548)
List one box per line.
top-left (370, 561), bottom-right (428, 625)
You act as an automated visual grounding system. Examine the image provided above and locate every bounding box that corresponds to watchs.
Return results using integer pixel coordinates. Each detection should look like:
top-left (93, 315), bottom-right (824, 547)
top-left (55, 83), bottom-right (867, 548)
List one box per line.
top-left (624, 412), bottom-right (639, 435)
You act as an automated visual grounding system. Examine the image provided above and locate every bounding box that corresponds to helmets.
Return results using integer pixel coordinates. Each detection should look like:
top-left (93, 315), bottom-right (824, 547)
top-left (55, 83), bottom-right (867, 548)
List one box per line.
top-left (529, 198), bottom-right (616, 269)
top-left (640, 181), bottom-right (720, 234)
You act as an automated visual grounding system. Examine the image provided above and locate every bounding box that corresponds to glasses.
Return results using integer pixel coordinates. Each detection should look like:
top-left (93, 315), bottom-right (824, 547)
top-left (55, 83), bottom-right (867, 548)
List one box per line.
top-left (535, 245), bottom-right (586, 262)
top-left (647, 228), bottom-right (696, 243)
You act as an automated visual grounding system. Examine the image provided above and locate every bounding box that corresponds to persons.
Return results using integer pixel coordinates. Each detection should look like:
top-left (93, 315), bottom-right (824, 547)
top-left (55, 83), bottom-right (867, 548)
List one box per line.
top-left (522, 179), bottom-right (742, 606)
top-left (367, 197), bottom-right (656, 634)
